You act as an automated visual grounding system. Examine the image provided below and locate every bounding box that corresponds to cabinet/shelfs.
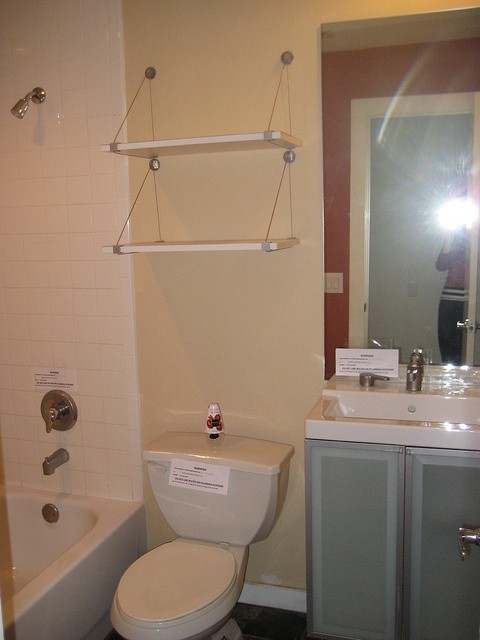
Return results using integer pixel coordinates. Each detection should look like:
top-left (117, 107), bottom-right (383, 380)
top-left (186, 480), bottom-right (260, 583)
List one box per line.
top-left (99, 51), bottom-right (299, 257)
top-left (303, 438), bottom-right (480, 640)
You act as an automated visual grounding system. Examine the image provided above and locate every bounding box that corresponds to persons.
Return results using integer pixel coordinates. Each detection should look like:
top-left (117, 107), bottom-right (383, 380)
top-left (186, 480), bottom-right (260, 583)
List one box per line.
top-left (433, 191), bottom-right (469, 367)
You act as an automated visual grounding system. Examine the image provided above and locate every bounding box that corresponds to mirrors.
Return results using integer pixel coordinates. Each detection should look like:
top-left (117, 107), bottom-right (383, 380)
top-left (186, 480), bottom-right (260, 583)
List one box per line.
top-left (322, 6), bottom-right (480, 382)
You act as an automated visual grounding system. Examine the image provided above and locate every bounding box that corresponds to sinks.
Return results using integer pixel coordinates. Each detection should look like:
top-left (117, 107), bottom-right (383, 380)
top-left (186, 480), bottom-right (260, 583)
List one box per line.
top-left (325, 396), bottom-right (479, 424)
top-left (109, 432), bottom-right (297, 639)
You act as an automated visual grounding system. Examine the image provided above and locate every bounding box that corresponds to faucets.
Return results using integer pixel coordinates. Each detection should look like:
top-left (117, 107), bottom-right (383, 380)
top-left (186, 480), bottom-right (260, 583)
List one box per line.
top-left (406, 348), bottom-right (424, 391)
top-left (41, 449), bottom-right (70, 477)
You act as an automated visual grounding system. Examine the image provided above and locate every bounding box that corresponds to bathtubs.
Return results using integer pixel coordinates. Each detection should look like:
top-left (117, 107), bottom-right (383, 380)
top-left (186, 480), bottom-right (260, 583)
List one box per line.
top-left (1, 486), bottom-right (144, 640)
